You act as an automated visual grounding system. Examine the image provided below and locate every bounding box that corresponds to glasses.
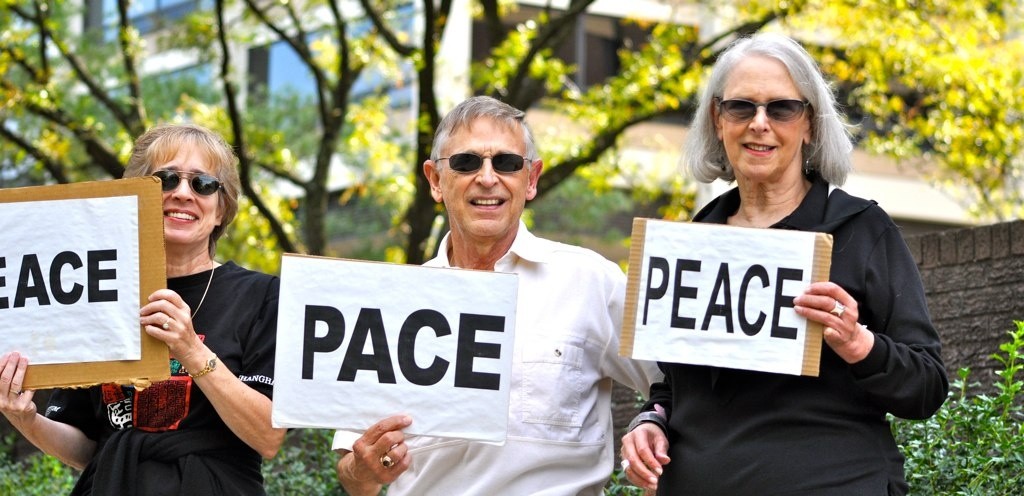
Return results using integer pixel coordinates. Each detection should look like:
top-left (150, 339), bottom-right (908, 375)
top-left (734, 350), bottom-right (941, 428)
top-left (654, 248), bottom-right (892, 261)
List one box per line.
top-left (716, 96), bottom-right (811, 124)
top-left (434, 153), bottom-right (531, 175)
top-left (151, 170), bottom-right (226, 197)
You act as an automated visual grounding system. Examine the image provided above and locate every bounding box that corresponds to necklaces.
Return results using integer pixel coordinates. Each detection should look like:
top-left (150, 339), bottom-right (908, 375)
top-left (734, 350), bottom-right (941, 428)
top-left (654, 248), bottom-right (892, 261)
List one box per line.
top-left (191, 259), bottom-right (214, 320)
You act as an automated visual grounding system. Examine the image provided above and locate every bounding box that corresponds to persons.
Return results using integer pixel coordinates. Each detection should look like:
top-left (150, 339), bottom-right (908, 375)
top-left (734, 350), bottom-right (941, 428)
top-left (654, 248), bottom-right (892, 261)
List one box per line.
top-left (0, 124), bottom-right (286, 496)
top-left (619, 32), bottom-right (949, 496)
top-left (331, 96), bottom-right (664, 496)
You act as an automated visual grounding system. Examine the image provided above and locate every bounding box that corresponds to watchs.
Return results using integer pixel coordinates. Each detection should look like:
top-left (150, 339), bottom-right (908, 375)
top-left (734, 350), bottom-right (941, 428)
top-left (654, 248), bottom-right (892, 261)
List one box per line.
top-left (187, 354), bottom-right (217, 378)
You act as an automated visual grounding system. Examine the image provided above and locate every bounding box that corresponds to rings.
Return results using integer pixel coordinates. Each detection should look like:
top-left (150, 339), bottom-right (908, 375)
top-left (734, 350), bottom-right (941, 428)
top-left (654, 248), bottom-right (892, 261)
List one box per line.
top-left (620, 460), bottom-right (629, 472)
top-left (379, 455), bottom-right (395, 469)
top-left (9, 388), bottom-right (22, 396)
top-left (162, 317), bottom-right (170, 330)
top-left (830, 300), bottom-right (845, 317)
